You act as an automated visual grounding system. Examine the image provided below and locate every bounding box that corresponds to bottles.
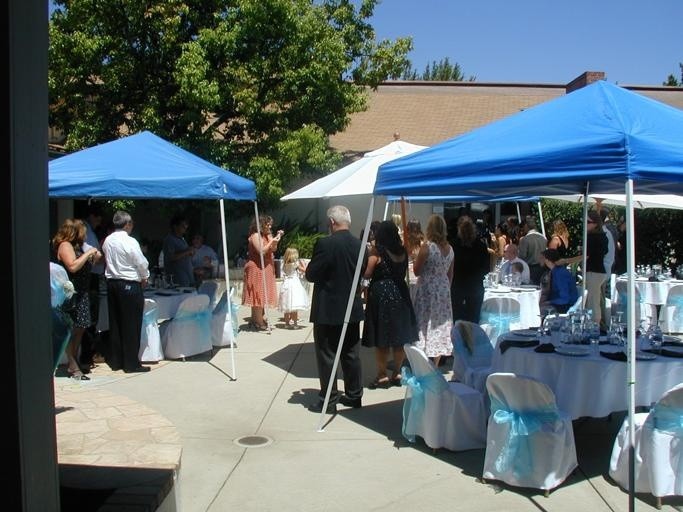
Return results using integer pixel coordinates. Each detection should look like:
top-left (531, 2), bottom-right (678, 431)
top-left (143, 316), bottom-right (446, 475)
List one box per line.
top-left (635, 263), bottom-right (673, 280)
top-left (149, 271), bottom-right (176, 290)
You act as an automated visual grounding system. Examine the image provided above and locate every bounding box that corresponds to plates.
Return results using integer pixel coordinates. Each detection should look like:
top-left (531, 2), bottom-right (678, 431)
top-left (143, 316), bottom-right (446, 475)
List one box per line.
top-left (513, 328), bottom-right (681, 362)
top-left (143, 287), bottom-right (199, 298)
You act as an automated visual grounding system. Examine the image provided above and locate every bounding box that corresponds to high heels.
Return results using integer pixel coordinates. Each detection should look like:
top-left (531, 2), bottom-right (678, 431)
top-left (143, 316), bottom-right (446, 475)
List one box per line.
top-left (391, 372), bottom-right (402, 388)
top-left (368, 375), bottom-right (390, 389)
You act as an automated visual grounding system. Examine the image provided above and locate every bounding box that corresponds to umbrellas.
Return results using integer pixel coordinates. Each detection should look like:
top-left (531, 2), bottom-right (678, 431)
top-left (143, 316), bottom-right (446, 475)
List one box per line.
top-left (280, 131), bottom-right (430, 283)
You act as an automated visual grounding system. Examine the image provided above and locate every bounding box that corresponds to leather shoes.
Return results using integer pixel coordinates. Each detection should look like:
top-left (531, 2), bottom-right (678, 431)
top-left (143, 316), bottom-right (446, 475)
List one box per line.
top-left (67, 364), bottom-right (150, 380)
top-left (308, 401), bottom-right (337, 414)
top-left (339, 396), bottom-right (362, 408)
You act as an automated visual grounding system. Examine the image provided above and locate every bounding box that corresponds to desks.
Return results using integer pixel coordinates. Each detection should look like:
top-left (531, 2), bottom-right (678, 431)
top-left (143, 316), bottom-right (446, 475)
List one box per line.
top-left (489, 324), bottom-right (682, 420)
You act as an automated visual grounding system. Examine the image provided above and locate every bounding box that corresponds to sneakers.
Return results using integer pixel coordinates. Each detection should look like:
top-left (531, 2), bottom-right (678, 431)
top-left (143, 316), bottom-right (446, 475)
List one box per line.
top-left (252, 322), bottom-right (300, 331)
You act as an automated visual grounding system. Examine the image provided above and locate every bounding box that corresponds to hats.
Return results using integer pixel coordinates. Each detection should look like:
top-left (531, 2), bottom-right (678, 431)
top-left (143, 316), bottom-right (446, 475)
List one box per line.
top-left (540, 248), bottom-right (559, 263)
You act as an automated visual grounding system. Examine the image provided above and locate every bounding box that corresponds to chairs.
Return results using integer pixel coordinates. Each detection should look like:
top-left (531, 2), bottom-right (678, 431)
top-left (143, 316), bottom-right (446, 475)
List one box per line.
top-left (52, 277), bottom-right (238, 364)
top-left (400, 344), bottom-right (487, 455)
top-left (481, 271), bottom-right (682, 336)
top-left (481, 372), bottom-right (579, 497)
top-left (608, 381), bottom-right (682, 511)
top-left (450, 319), bottom-right (495, 392)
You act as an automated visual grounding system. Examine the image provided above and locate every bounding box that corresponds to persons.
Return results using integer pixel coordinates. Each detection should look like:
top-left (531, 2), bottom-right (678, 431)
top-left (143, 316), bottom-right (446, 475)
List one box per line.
top-left (305, 207), bottom-right (486, 413)
top-left (487, 208), bottom-right (626, 327)
top-left (162, 214), bottom-right (218, 286)
top-left (51, 208), bottom-right (151, 380)
top-left (243, 214), bottom-right (310, 332)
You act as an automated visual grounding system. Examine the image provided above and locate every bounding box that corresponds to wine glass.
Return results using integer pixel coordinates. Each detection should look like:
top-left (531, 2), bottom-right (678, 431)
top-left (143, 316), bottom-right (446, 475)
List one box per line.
top-left (535, 304), bottom-right (665, 353)
top-left (483, 271), bottom-right (523, 288)
